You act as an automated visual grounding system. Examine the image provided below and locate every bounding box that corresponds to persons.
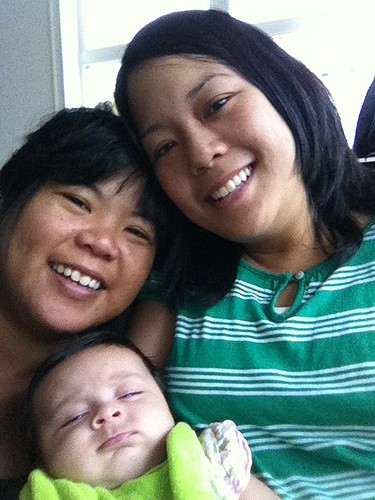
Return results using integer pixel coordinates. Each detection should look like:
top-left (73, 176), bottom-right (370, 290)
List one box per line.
top-left (0, 99), bottom-right (188, 499)
top-left (114, 8), bottom-right (374, 500)
top-left (17, 331), bottom-right (286, 499)
